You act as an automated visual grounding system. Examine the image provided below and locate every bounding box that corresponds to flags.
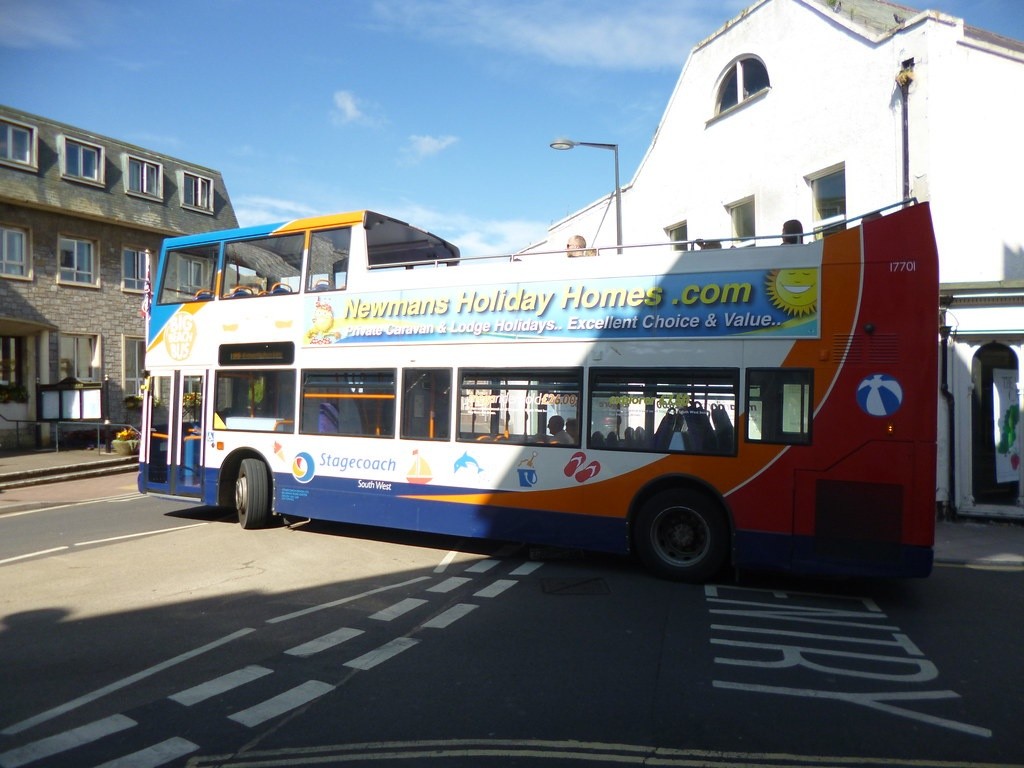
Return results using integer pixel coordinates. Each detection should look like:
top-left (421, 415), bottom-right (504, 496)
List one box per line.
top-left (142, 254), bottom-right (152, 320)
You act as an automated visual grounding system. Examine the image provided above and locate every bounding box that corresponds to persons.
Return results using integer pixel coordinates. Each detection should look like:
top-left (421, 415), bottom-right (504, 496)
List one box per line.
top-left (585, 249), bottom-right (599, 256)
top-left (535, 415), bottom-right (576, 444)
top-left (781, 220), bottom-right (803, 244)
top-left (695, 239), bottom-right (722, 250)
top-left (567, 235), bottom-right (586, 256)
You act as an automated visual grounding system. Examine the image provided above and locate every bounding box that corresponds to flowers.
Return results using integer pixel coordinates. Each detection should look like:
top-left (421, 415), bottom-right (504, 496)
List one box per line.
top-left (117, 426), bottom-right (141, 440)
top-left (183, 392), bottom-right (202, 407)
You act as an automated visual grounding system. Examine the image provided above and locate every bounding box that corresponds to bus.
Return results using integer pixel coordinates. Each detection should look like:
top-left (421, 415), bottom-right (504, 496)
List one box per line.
top-left (143, 198), bottom-right (941, 580)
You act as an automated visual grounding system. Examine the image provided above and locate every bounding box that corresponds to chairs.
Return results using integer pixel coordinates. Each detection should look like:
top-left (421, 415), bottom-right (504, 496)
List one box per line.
top-left (477, 400), bottom-right (735, 450)
top-left (196, 280), bottom-right (332, 300)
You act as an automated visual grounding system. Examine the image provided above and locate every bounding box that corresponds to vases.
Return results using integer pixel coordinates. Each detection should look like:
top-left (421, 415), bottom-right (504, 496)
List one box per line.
top-left (112, 439), bottom-right (140, 455)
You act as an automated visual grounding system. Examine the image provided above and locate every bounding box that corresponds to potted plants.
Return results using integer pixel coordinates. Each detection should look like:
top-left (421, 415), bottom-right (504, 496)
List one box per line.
top-left (123, 396), bottom-right (160, 411)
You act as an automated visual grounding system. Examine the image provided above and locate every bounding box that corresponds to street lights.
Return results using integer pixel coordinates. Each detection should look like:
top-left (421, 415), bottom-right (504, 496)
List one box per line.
top-left (548, 141), bottom-right (622, 259)
